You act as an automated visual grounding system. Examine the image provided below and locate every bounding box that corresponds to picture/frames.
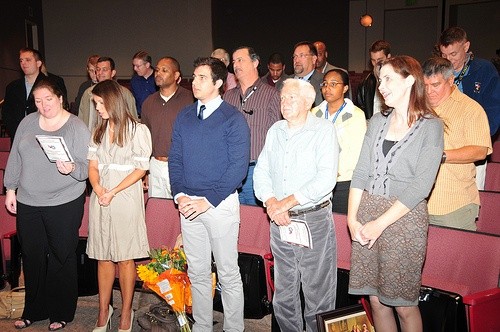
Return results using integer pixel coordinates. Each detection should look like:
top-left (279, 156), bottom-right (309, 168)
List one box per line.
top-left (315, 301), bottom-right (401, 332)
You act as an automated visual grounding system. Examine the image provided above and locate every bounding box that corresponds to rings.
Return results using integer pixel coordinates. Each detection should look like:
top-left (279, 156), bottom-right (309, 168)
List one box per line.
top-left (356, 236), bottom-right (361, 240)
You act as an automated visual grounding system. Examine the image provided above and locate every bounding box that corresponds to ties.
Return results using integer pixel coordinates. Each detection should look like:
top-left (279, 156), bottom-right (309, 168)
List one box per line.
top-left (299, 77), bottom-right (303, 80)
top-left (198, 105), bottom-right (206, 121)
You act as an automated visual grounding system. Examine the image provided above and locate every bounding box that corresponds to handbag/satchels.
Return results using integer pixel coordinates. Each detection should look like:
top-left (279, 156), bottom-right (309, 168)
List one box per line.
top-left (0, 285), bottom-right (25, 319)
top-left (416, 282), bottom-right (471, 332)
top-left (138, 302), bottom-right (195, 332)
top-left (74, 236), bottom-right (99, 297)
top-left (210, 250), bottom-right (268, 319)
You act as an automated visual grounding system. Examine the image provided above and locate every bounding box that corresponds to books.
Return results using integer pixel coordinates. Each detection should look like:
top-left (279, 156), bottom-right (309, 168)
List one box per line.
top-left (279, 219), bottom-right (313, 250)
top-left (36, 134), bottom-right (74, 164)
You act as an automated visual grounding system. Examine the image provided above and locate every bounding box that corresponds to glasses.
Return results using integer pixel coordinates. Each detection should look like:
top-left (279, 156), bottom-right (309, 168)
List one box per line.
top-left (133, 63), bottom-right (144, 68)
top-left (293, 53), bottom-right (314, 59)
top-left (319, 80), bottom-right (347, 87)
top-left (95, 68), bottom-right (110, 72)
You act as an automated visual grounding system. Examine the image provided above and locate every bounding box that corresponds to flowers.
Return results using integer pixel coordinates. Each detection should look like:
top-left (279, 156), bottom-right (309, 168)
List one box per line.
top-left (137, 246), bottom-right (190, 332)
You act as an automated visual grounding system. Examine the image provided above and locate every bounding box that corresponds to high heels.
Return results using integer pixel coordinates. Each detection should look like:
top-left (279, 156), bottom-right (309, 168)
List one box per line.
top-left (117, 308), bottom-right (135, 332)
top-left (92, 304), bottom-right (114, 332)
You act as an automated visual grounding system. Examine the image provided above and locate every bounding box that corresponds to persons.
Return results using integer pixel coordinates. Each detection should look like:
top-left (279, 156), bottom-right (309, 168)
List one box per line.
top-left (169, 57), bottom-right (338, 332)
top-left (422, 26), bottom-right (500, 231)
top-left (222, 46), bottom-right (280, 206)
top-left (141, 57), bottom-right (194, 199)
top-left (71, 54), bottom-right (101, 116)
top-left (41, 57), bottom-right (67, 98)
top-left (78, 57), bottom-right (116, 132)
top-left (4, 75), bottom-right (92, 331)
top-left (4, 46), bottom-right (66, 149)
top-left (86, 80), bottom-right (152, 332)
top-left (253, 55), bottom-right (452, 332)
top-left (130, 50), bottom-right (160, 119)
top-left (282, 40), bottom-right (391, 118)
top-left (212, 48), bottom-right (237, 93)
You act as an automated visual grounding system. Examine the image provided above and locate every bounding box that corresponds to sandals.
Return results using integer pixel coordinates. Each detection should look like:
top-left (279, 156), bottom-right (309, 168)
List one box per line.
top-left (48, 320), bottom-right (68, 330)
top-left (14, 318), bottom-right (35, 329)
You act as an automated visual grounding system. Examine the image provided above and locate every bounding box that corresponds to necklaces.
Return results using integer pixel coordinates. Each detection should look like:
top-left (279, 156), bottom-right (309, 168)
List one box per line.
top-left (393, 113), bottom-right (409, 143)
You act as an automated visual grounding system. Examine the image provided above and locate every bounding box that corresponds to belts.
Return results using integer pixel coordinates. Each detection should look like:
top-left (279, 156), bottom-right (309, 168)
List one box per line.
top-left (249, 162), bottom-right (256, 166)
top-left (288, 197), bottom-right (332, 217)
top-left (154, 156), bottom-right (169, 161)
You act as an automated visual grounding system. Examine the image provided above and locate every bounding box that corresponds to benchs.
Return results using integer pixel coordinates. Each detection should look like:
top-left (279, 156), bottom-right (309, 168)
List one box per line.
top-left (0, 133), bottom-right (500, 332)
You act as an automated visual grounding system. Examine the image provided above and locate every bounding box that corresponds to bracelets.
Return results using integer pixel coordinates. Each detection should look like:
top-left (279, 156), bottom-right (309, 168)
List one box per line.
top-left (110, 191), bottom-right (116, 197)
top-left (440, 152), bottom-right (446, 162)
top-left (6, 190), bottom-right (15, 193)
